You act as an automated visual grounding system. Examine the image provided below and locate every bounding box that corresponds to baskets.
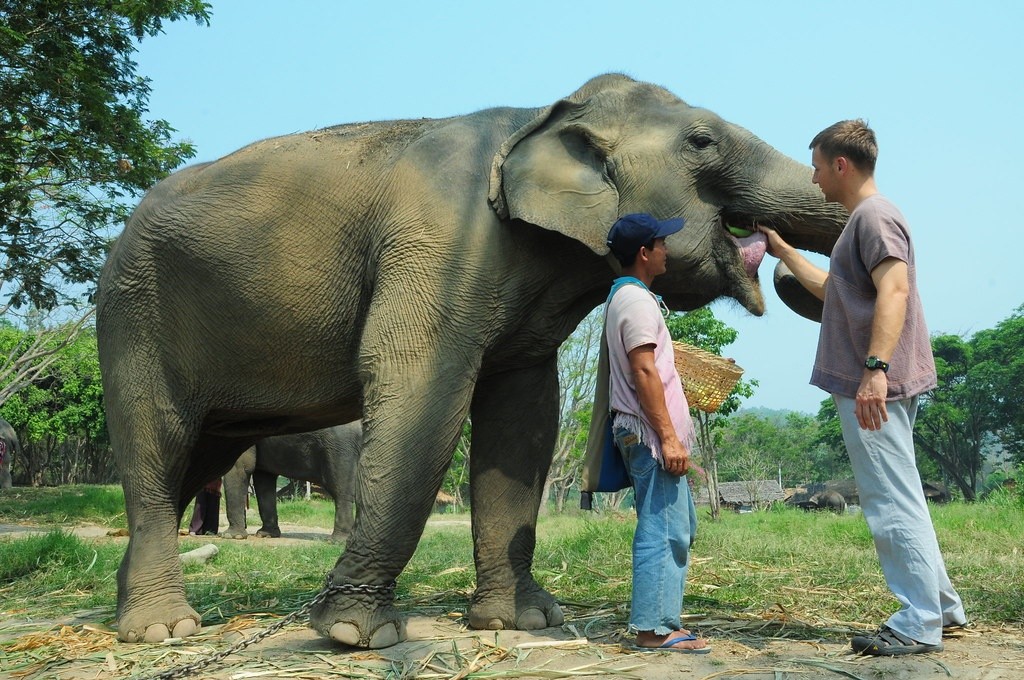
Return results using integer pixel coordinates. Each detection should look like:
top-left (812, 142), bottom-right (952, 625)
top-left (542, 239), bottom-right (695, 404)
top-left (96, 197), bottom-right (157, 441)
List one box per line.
top-left (672, 340), bottom-right (745, 413)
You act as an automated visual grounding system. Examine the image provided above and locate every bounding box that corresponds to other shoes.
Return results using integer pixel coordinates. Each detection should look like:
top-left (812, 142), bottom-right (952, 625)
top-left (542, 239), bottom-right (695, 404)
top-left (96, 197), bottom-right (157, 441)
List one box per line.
top-left (852, 625), bottom-right (944, 655)
top-left (943, 617), bottom-right (967, 637)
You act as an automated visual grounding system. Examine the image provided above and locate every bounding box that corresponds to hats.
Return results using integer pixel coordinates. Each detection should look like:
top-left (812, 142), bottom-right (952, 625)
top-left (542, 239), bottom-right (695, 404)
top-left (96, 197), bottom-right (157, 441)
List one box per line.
top-left (606, 212), bottom-right (685, 254)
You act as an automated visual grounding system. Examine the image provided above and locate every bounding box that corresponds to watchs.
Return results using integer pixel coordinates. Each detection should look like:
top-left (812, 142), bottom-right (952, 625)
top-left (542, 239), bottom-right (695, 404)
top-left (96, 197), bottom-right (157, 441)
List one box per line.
top-left (864, 356), bottom-right (889, 373)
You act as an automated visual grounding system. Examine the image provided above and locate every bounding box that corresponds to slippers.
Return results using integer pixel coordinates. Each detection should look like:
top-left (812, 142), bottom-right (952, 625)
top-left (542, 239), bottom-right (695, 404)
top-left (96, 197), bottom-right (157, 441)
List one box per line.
top-left (630, 622), bottom-right (711, 654)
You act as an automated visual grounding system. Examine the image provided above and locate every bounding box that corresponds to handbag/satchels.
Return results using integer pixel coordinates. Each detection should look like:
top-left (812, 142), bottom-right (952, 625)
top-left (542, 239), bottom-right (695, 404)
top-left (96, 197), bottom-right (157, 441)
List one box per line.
top-left (580, 329), bottom-right (632, 510)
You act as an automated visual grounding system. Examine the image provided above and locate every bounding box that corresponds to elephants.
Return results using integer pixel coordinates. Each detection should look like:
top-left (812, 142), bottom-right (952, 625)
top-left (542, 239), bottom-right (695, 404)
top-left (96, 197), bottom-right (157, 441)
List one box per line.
top-left (96, 70), bottom-right (853, 648)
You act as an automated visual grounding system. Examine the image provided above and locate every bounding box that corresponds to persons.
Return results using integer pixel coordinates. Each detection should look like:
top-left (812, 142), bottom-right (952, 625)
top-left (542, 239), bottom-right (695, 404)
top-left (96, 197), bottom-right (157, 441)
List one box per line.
top-left (607, 213), bottom-right (710, 654)
top-left (188, 477), bottom-right (222, 536)
top-left (747, 118), bottom-right (968, 655)
